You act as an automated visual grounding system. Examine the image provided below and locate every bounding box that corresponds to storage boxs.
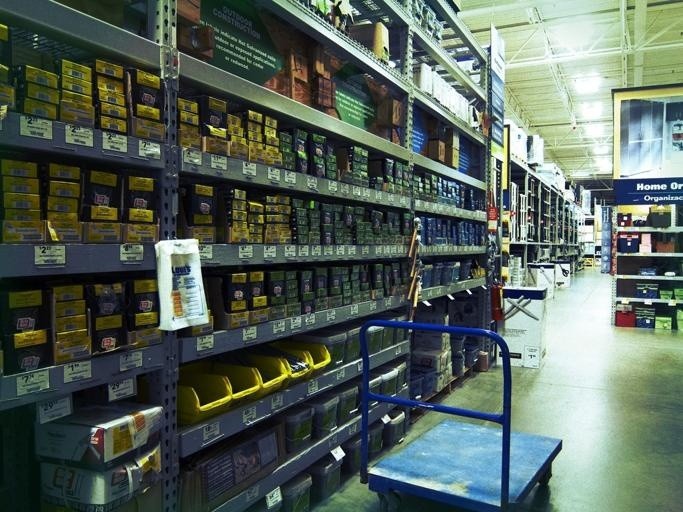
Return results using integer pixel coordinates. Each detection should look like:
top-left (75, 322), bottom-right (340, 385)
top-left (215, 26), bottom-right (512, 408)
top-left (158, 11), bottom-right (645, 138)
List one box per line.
top-left (496, 287), bottom-right (549, 367)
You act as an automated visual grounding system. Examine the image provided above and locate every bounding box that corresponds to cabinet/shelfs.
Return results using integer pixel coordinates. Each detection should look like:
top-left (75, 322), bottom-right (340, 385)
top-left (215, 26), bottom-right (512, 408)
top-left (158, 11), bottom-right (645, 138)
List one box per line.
top-left (3, 0), bottom-right (503, 511)
top-left (502, 119), bottom-right (588, 300)
top-left (611, 193), bottom-right (683, 331)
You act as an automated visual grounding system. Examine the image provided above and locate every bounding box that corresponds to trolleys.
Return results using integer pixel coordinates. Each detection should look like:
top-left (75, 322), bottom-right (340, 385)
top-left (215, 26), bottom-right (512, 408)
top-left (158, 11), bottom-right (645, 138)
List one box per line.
top-left (359, 319), bottom-right (562, 508)
